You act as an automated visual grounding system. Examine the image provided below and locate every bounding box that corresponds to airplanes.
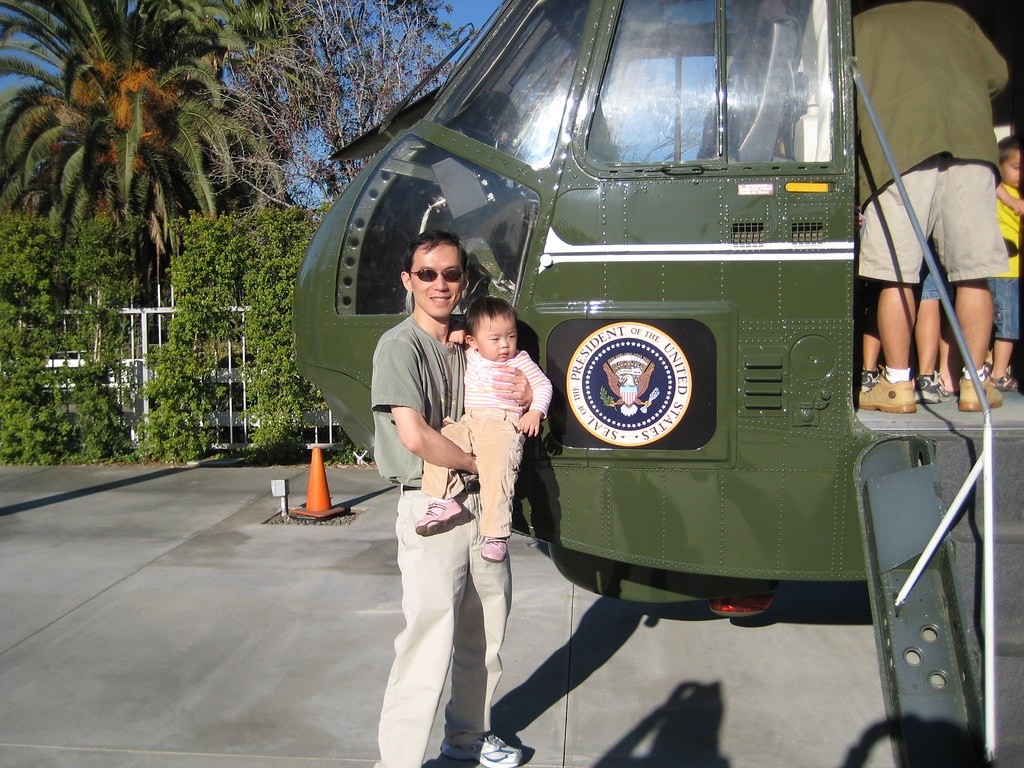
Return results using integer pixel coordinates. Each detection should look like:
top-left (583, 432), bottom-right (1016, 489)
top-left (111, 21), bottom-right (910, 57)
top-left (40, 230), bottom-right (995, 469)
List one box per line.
top-left (290, 0), bottom-right (1024, 768)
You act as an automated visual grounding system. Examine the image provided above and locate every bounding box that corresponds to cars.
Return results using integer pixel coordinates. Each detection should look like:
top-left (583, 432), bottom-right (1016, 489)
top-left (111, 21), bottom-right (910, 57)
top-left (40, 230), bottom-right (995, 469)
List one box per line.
top-left (34, 354), bottom-right (142, 391)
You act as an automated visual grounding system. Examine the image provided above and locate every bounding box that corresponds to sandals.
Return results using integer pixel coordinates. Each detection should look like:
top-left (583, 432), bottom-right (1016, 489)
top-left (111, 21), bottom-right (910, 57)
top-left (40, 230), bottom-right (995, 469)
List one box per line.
top-left (990, 375), bottom-right (1023, 392)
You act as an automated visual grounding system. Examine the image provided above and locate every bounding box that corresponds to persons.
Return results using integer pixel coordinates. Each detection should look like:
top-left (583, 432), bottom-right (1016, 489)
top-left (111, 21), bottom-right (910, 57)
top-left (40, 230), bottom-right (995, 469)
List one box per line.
top-left (696, 0), bottom-right (1024, 414)
top-left (406, 297), bottom-right (552, 560)
top-left (371, 231), bottom-right (523, 768)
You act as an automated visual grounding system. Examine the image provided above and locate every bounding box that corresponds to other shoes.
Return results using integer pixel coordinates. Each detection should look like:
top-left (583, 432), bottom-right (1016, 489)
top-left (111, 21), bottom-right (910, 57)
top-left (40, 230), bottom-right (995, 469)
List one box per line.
top-left (481, 536), bottom-right (509, 562)
top-left (415, 499), bottom-right (462, 536)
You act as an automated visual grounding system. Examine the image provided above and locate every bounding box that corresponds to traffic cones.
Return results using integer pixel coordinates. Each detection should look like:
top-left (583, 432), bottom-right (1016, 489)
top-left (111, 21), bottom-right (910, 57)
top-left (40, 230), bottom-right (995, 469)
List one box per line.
top-left (289, 448), bottom-right (349, 521)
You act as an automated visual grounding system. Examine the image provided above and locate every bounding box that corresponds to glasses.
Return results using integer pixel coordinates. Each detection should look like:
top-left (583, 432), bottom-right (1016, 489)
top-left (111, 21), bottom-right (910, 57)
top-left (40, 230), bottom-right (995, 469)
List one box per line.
top-left (411, 269), bottom-right (466, 282)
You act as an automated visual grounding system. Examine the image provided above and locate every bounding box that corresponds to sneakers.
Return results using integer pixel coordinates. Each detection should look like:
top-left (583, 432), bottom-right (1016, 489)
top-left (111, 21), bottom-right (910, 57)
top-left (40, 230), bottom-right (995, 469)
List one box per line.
top-left (440, 733), bottom-right (523, 768)
top-left (855, 364), bottom-right (917, 414)
top-left (860, 369), bottom-right (879, 393)
top-left (915, 371), bottom-right (958, 404)
top-left (959, 376), bottom-right (1003, 412)
